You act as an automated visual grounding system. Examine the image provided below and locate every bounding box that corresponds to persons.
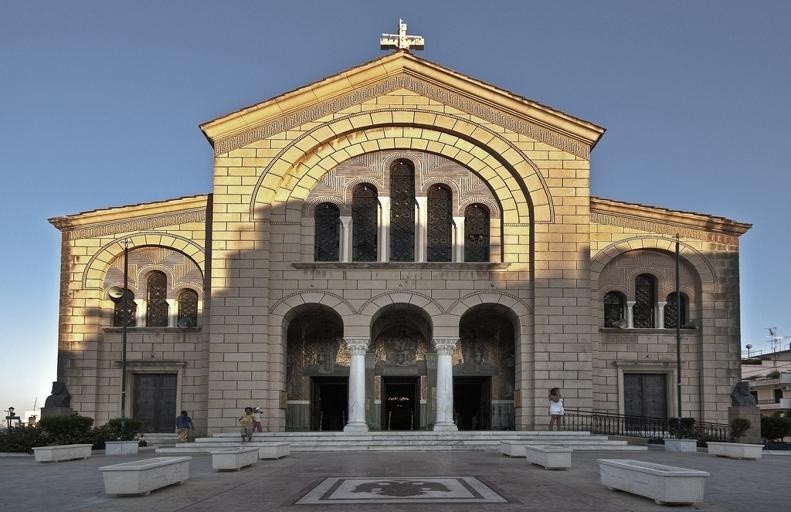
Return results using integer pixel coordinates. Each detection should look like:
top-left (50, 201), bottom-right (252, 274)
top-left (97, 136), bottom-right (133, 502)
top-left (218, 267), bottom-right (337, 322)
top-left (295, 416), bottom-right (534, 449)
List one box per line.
top-left (548, 387), bottom-right (565, 430)
top-left (174, 409), bottom-right (194, 442)
top-left (239, 406), bottom-right (257, 442)
top-left (251, 407), bottom-right (265, 432)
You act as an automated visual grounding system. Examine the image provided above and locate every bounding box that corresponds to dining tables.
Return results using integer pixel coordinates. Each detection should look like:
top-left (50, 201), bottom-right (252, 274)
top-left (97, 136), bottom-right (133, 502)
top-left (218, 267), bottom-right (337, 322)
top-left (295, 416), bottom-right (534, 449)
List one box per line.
top-left (662, 417), bottom-right (699, 454)
top-left (31, 408), bottom-right (96, 463)
top-left (704, 417), bottom-right (766, 460)
top-left (99, 415), bottom-right (146, 456)
